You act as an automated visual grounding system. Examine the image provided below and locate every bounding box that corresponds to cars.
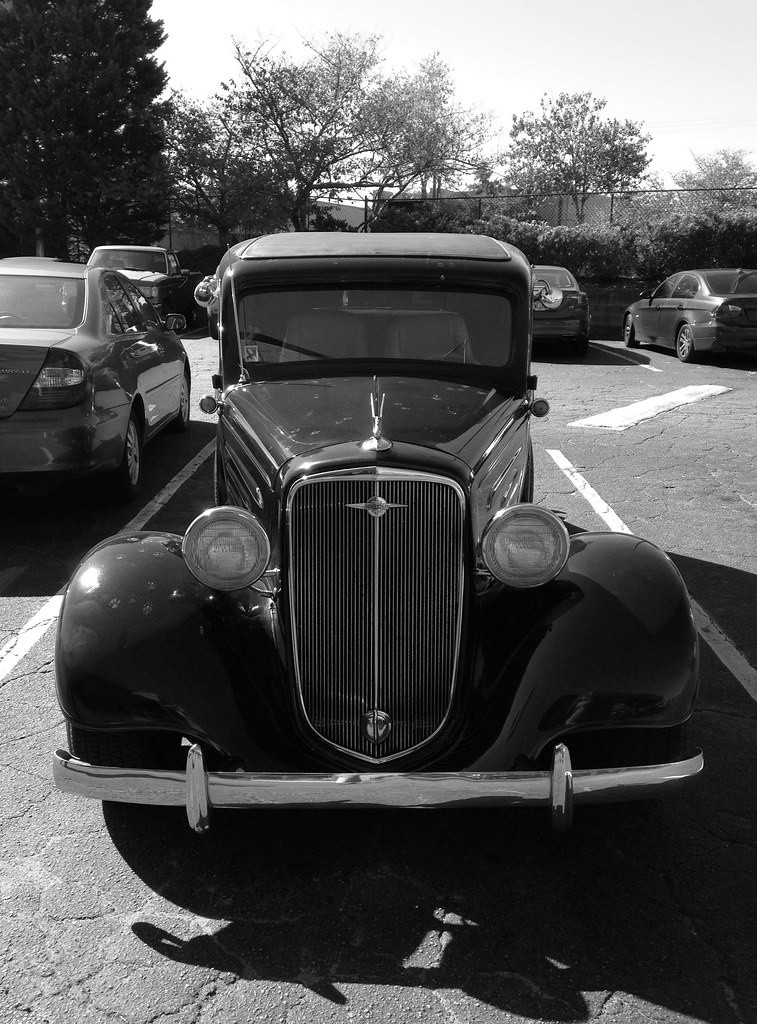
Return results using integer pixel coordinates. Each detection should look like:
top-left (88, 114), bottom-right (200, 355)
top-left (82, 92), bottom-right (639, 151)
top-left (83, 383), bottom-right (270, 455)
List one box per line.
top-left (59, 244), bottom-right (204, 328)
top-left (0, 254), bottom-right (193, 504)
top-left (531, 263), bottom-right (591, 356)
top-left (621, 268), bottom-right (757, 363)
top-left (49, 230), bottom-right (709, 833)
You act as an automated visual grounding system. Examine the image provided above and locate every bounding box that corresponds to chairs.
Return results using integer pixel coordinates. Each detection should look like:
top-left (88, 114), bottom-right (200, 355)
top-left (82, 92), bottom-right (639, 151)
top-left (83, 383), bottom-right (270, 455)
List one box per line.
top-left (277, 312), bottom-right (366, 364)
top-left (392, 312), bottom-right (483, 366)
